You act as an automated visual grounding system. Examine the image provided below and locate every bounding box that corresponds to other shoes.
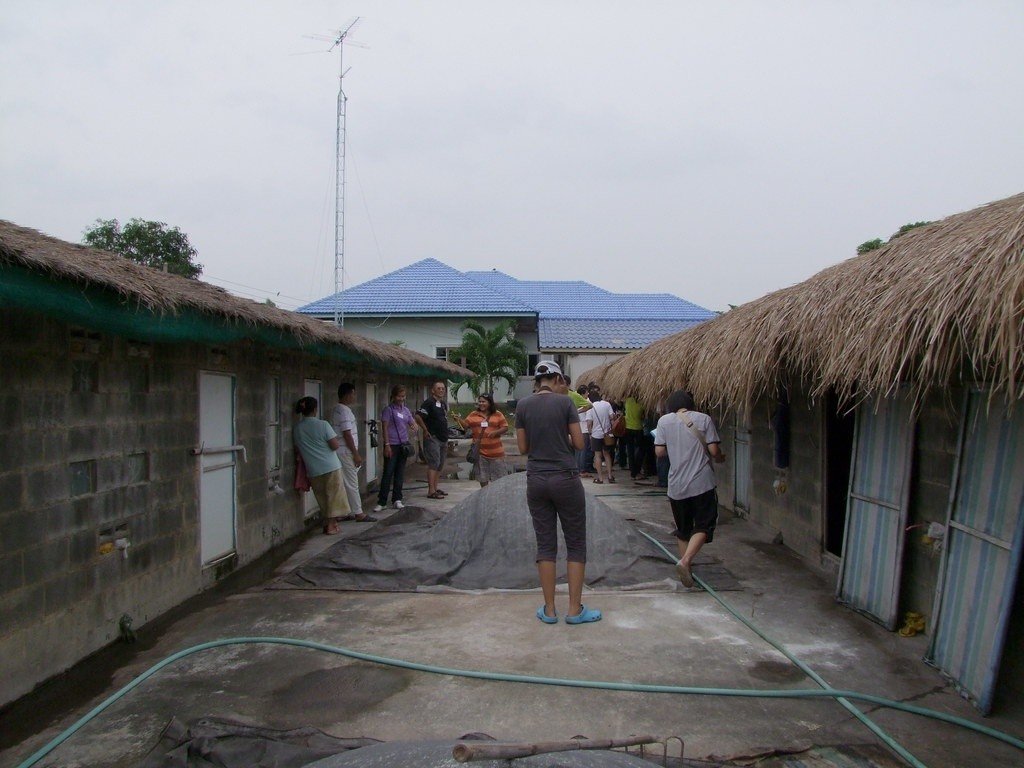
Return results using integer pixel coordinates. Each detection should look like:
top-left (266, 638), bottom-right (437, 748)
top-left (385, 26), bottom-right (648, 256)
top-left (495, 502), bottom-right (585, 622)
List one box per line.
top-left (579, 473), bottom-right (593, 477)
top-left (589, 468), bottom-right (598, 473)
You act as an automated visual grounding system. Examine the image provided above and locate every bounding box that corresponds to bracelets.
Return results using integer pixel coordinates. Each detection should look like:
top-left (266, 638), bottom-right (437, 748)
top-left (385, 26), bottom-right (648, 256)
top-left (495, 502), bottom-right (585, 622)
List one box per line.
top-left (385, 442), bottom-right (390, 446)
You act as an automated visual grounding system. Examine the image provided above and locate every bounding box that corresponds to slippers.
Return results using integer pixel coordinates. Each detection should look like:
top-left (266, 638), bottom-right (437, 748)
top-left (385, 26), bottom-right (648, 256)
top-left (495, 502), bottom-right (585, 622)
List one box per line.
top-left (325, 520), bottom-right (338, 528)
top-left (536, 604), bottom-right (558, 623)
top-left (593, 478), bottom-right (603, 484)
top-left (356, 514), bottom-right (378, 522)
top-left (676, 560), bottom-right (693, 588)
top-left (436, 489), bottom-right (448, 496)
top-left (566, 603), bottom-right (602, 624)
top-left (427, 491), bottom-right (444, 499)
top-left (608, 476), bottom-right (615, 482)
top-left (326, 527), bottom-right (340, 535)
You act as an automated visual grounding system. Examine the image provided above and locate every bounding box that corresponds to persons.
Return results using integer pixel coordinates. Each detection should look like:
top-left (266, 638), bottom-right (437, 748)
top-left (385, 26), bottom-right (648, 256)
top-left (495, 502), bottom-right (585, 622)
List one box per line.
top-left (575, 381), bottom-right (668, 487)
top-left (654, 389), bottom-right (727, 592)
top-left (453, 393), bottom-right (509, 488)
top-left (373, 384), bottom-right (419, 512)
top-left (415, 381), bottom-right (449, 499)
top-left (515, 361), bottom-right (601, 624)
top-left (329, 383), bottom-right (378, 522)
top-left (558, 374), bottom-right (593, 414)
top-left (294, 396), bottom-right (351, 535)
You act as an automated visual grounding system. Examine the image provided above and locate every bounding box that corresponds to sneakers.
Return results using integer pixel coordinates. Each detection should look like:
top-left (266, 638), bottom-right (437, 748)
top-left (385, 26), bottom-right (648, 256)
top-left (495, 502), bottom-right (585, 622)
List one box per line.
top-left (393, 500), bottom-right (405, 509)
top-left (374, 505), bottom-right (387, 512)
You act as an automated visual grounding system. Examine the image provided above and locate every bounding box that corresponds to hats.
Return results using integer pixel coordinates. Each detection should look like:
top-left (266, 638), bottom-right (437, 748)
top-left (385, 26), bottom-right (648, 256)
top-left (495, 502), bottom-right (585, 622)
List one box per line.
top-left (534, 360), bottom-right (566, 385)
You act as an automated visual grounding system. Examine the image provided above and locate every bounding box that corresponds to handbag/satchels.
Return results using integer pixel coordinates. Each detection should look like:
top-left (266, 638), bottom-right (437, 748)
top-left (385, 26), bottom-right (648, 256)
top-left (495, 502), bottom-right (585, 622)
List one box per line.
top-left (466, 440), bottom-right (481, 462)
top-left (604, 433), bottom-right (615, 446)
top-left (401, 444), bottom-right (415, 458)
